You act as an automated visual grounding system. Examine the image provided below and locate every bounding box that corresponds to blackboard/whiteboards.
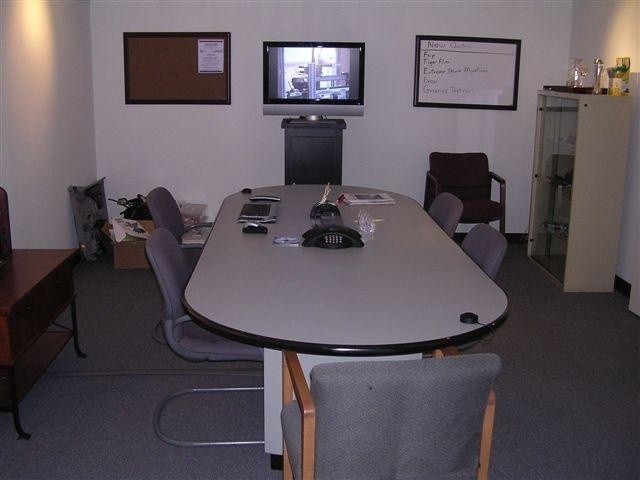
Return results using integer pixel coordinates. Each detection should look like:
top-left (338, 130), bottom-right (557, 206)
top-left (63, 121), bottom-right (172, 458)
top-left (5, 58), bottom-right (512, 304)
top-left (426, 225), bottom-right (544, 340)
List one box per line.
top-left (413, 35), bottom-right (521, 111)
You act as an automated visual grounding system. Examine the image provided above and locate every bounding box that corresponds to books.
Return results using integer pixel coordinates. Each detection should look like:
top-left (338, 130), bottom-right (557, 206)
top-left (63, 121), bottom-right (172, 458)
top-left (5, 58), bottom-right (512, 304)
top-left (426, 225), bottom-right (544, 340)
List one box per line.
top-left (340, 193), bottom-right (396, 205)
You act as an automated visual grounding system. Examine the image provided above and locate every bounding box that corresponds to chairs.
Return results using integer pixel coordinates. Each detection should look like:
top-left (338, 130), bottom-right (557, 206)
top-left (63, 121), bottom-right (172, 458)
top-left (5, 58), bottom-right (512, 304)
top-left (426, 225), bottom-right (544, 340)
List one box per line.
top-left (279, 344), bottom-right (505, 480)
top-left (460, 222), bottom-right (509, 281)
top-left (535, 153), bottom-right (575, 266)
top-left (425, 192), bottom-right (465, 238)
top-left (147, 187), bottom-right (215, 346)
top-left (143, 228), bottom-right (265, 447)
top-left (422, 150), bottom-right (508, 234)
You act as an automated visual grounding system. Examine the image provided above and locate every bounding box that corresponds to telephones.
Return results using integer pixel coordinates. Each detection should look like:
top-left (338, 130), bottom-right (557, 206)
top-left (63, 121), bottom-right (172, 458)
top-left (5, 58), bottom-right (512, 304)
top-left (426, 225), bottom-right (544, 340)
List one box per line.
top-left (302, 225), bottom-right (364, 248)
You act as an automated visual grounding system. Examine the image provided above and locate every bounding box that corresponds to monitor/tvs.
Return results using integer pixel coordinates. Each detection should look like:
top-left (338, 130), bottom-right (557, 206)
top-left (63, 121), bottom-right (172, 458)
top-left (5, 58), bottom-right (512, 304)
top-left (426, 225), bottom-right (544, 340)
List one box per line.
top-left (261, 41), bottom-right (366, 122)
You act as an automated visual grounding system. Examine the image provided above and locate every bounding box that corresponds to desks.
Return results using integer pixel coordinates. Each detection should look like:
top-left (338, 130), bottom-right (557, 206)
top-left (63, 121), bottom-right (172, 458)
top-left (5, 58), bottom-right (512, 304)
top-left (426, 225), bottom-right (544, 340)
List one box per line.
top-left (179, 183), bottom-right (512, 473)
top-left (0, 246), bottom-right (90, 441)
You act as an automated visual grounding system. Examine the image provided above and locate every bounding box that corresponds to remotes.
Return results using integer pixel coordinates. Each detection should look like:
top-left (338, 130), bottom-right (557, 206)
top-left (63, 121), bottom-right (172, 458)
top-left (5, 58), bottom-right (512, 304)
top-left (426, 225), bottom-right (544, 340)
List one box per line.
top-left (248, 196), bottom-right (281, 201)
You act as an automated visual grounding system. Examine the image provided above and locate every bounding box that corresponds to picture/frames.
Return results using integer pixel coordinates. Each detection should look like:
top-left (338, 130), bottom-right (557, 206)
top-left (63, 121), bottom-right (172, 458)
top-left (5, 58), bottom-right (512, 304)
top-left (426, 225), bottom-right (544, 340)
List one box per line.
top-left (122, 31), bottom-right (231, 106)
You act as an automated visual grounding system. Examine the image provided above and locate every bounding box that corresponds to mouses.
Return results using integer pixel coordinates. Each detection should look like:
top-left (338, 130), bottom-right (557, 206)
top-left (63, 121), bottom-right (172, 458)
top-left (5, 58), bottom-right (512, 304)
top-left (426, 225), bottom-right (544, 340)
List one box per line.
top-left (241, 222), bottom-right (268, 234)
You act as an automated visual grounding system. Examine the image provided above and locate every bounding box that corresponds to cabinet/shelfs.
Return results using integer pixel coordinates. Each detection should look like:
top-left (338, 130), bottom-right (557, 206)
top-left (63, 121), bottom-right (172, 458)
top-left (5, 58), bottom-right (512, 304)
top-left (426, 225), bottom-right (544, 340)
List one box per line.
top-left (526, 89), bottom-right (634, 294)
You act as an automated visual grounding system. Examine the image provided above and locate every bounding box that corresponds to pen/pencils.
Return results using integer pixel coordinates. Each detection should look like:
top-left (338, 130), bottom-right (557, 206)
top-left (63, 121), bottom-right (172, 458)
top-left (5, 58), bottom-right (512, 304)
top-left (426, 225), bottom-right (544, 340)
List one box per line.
top-left (355, 219), bottom-right (382, 222)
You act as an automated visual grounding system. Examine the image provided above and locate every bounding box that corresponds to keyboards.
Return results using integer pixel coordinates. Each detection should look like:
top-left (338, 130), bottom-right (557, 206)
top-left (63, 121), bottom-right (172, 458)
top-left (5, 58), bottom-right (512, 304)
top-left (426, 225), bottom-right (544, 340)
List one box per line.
top-left (238, 203), bottom-right (279, 223)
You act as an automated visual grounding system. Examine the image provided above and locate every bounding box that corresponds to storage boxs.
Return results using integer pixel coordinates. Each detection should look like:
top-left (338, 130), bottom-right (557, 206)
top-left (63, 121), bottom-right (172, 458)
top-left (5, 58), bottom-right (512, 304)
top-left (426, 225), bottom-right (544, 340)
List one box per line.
top-left (101, 218), bottom-right (154, 271)
